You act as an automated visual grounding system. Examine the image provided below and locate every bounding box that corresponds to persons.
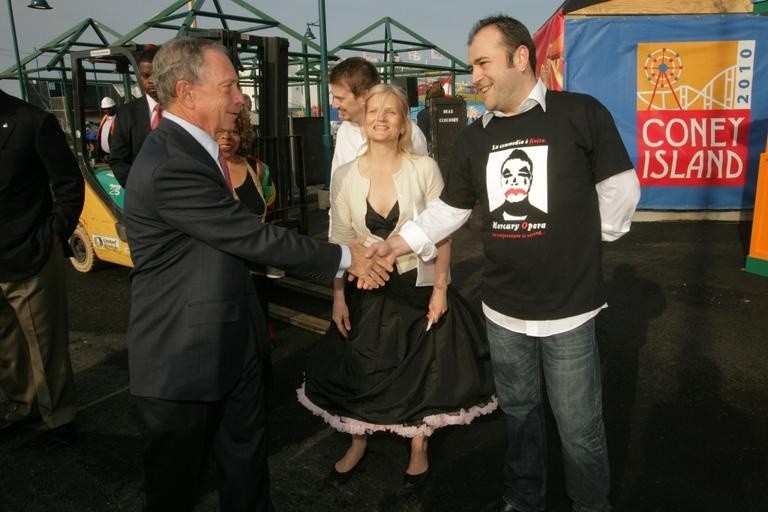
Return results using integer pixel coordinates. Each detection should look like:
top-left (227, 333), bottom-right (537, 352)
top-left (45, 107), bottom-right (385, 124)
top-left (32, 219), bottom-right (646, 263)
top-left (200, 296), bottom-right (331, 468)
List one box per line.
top-left (349, 16), bottom-right (642, 511)
top-left (287, 84), bottom-right (501, 488)
top-left (416, 84), bottom-right (446, 151)
top-left (0, 87), bottom-right (84, 454)
top-left (97, 96), bottom-right (118, 158)
top-left (123, 36), bottom-right (394, 511)
top-left (326, 58), bottom-right (423, 249)
top-left (211, 97), bottom-right (284, 352)
top-left (241, 94), bottom-right (261, 127)
top-left (109, 47), bottom-right (162, 191)
top-left (489, 149), bottom-right (546, 239)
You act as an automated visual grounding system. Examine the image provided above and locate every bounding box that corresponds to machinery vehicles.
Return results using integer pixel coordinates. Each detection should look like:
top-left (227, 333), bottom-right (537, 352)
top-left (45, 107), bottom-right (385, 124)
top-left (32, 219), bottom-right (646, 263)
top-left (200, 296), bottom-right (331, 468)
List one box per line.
top-left (68, 43), bottom-right (286, 279)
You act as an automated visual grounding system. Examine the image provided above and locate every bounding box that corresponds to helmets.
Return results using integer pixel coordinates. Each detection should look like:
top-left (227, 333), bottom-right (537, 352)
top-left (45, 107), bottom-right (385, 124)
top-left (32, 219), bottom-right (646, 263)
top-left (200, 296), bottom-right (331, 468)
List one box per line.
top-left (101, 96), bottom-right (116, 110)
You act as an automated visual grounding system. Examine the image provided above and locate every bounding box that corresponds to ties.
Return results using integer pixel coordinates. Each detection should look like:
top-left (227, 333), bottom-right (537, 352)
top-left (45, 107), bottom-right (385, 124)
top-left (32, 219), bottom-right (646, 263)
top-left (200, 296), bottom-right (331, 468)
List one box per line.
top-left (149, 103), bottom-right (162, 130)
top-left (216, 146), bottom-right (234, 199)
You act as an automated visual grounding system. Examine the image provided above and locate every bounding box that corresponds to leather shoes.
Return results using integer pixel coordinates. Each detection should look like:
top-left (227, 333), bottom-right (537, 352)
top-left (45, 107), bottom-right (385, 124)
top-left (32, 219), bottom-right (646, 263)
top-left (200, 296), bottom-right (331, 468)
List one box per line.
top-left (48, 426), bottom-right (75, 449)
top-left (402, 460), bottom-right (430, 489)
top-left (0, 416), bottom-right (35, 434)
top-left (328, 441), bottom-right (369, 483)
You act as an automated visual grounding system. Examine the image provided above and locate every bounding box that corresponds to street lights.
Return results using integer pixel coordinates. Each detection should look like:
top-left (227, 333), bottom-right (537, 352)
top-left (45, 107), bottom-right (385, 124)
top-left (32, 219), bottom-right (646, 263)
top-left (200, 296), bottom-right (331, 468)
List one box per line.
top-left (7, 1), bottom-right (53, 101)
top-left (305, 1), bottom-right (332, 187)
